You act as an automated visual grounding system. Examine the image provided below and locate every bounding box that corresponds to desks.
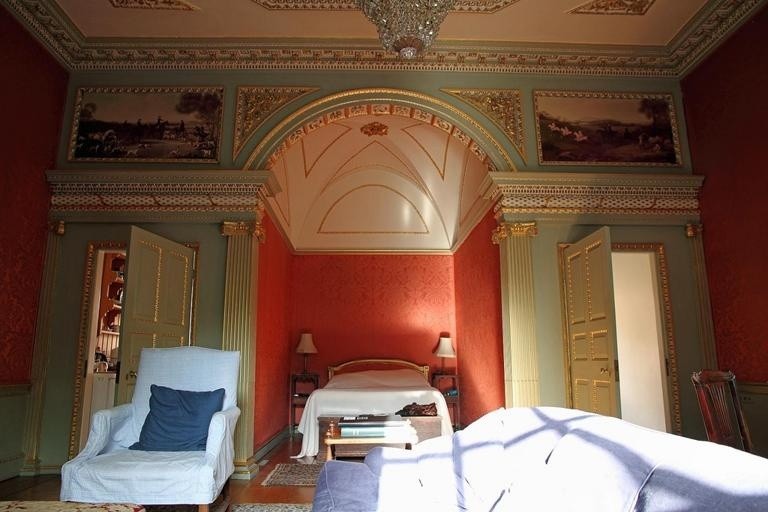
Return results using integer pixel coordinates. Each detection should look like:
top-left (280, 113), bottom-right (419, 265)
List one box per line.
top-left (323, 436), bottom-right (418, 462)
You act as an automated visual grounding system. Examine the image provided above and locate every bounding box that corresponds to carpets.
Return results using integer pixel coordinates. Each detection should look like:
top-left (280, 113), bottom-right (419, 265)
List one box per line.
top-left (260, 462), bottom-right (323, 487)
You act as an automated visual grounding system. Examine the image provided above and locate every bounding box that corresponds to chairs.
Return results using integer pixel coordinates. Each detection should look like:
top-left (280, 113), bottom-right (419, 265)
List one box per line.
top-left (691, 367), bottom-right (756, 454)
top-left (60, 344), bottom-right (246, 512)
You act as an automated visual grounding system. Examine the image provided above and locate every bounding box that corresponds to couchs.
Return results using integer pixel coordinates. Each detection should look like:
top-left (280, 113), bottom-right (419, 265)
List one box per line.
top-left (314, 405), bottom-right (768, 509)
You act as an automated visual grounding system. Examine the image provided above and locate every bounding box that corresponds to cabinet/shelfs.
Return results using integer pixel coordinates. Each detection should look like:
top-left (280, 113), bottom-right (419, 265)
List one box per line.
top-left (288, 367), bottom-right (320, 436)
top-left (430, 373), bottom-right (461, 432)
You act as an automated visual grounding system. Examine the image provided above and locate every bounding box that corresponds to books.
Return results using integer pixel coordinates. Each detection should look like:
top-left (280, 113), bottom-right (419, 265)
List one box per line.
top-left (340, 427), bottom-right (385, 438)
top-left (337, 415), bottom-right (404, 427)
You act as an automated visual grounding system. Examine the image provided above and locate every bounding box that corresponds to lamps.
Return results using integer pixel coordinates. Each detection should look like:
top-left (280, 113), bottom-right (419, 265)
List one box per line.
top-left (355, 0), bottom-right (459, 64)
top-left (432, 336), bottom-right (457, 372)
top-left (296, 333), bottom-right (318, 375)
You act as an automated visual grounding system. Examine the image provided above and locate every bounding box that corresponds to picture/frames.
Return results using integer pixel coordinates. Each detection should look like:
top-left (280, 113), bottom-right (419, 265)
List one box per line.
top-left (533, 86), bottom-right (686, 169)
top-left (66, 83), bottom-right (227, 165)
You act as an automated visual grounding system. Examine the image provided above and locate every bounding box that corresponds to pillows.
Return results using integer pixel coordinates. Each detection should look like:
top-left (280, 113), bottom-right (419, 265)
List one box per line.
top-left (124, 383), bottom-right (230, 454)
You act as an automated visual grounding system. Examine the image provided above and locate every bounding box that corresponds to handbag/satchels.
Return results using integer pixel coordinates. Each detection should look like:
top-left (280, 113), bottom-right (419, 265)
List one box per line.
top-left (394, 402), bottom-right (437, 416)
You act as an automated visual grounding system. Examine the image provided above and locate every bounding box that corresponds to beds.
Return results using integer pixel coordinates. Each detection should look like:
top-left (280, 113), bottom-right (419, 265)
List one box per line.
top-left (310, 356), bottom-right (452, 461)
